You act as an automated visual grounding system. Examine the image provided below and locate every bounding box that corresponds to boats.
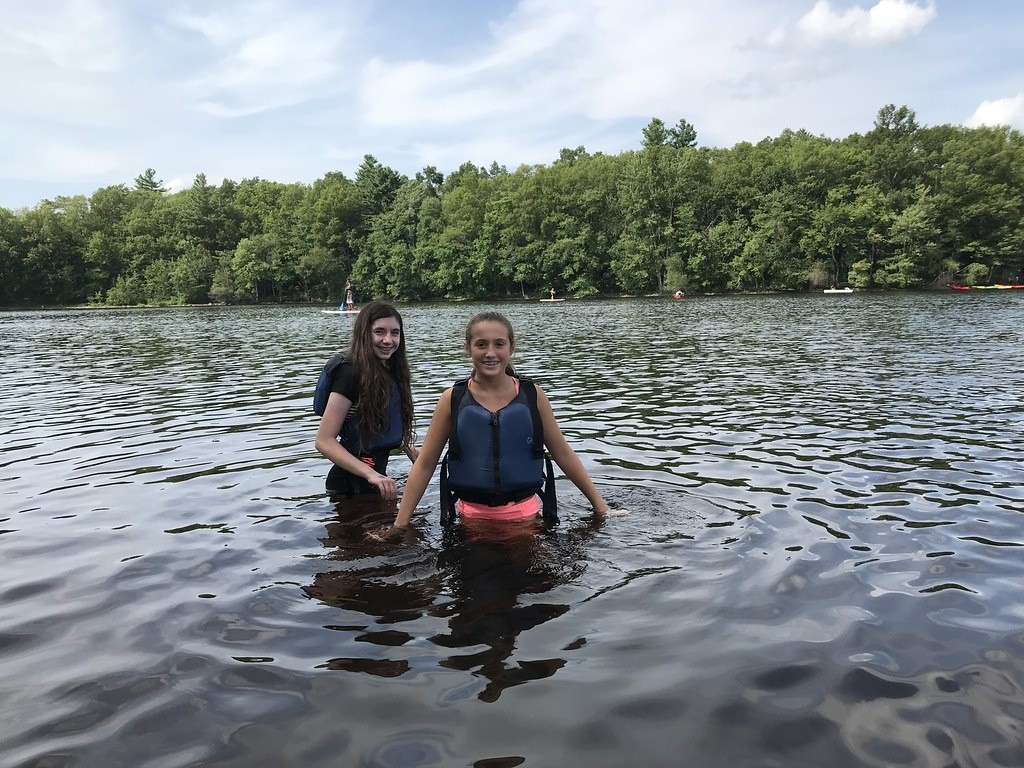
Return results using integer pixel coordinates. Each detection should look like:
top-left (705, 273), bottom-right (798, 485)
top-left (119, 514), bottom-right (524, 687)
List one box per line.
top-left (823, 287), bottom-right (853, 293)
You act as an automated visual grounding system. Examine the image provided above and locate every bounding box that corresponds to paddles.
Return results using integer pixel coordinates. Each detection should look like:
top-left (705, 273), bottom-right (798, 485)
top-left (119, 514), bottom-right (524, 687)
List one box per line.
top-left (339, 295), bottom-right (345, 311)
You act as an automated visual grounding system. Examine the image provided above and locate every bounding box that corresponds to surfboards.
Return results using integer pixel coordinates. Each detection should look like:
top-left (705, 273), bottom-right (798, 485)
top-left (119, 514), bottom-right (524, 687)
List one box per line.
top-left (321, 310), bottom-right (360, 314)
top-left (539, 298), bottom-right (565, 301)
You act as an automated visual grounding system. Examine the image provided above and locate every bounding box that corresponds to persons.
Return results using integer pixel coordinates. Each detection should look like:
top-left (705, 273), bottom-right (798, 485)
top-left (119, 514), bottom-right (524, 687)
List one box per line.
top-left (345, 281), bottom-right (354, 311)
top-left (314, 301), bottom-right (421, 501)
top-left (361, 312), bottom-right (608, 545)
top-left (550, 288), bottom-right (555, 300)
